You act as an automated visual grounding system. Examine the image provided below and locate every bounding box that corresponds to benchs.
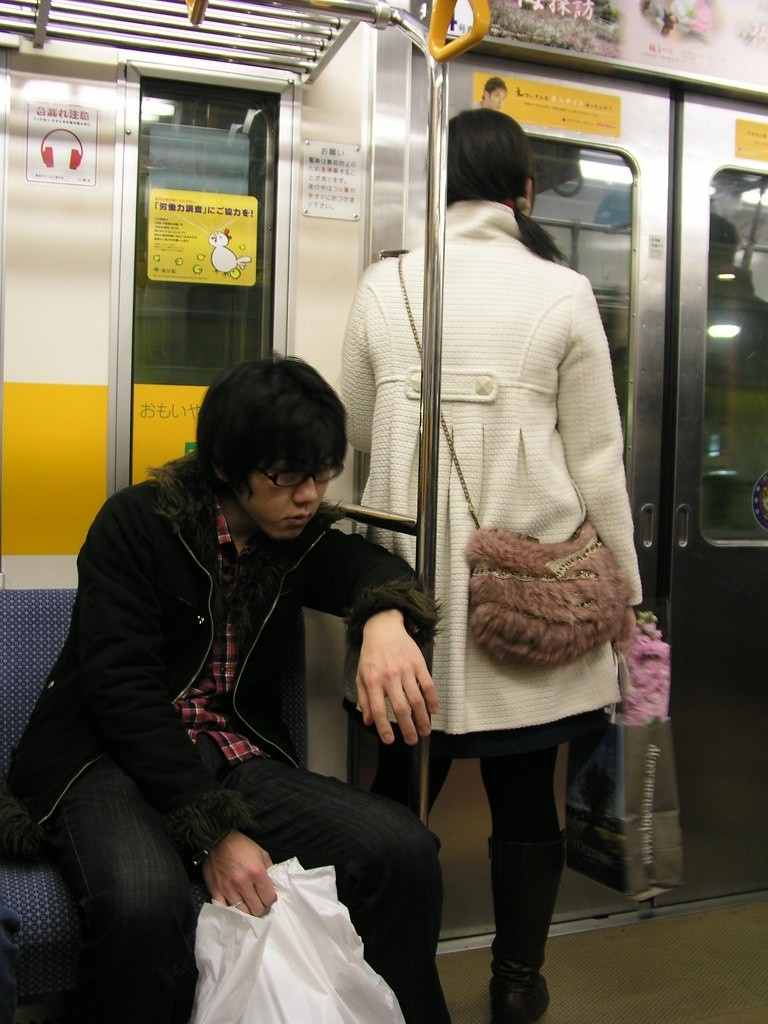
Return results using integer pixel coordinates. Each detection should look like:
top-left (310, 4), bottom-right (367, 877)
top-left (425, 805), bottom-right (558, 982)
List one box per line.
top-left (0, 589), bottom-right (307, 1024)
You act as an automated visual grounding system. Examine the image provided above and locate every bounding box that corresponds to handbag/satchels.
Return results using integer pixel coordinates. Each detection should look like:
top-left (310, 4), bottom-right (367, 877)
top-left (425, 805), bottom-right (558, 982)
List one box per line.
top-left (563, 714), bottom-right (686, 902)
top-left (193, 859), bottom-right (408, 1024)
top-left (467, 520), bottom-right (629, 668)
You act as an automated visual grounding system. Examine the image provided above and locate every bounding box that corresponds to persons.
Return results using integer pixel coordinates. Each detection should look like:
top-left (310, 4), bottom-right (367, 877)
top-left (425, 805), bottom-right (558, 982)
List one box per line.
top-left (7, 356), bottom-right (444, 1024)
top-left (340, 109), bottom-right (644, 1024)
top-left (473, 77), bottom-right (508, 111)
top-left (701, 214), bottom-right (768, 530)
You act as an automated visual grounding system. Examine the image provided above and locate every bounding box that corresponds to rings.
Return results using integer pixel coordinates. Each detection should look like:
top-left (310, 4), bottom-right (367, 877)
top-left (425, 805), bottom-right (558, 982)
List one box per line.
top-left (231, 900), bottom-right (243, 908)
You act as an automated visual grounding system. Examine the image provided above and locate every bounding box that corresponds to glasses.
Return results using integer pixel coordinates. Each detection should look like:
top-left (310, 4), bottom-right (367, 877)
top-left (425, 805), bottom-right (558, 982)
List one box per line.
top-left (255, 462), bottom-right (344, 489)
